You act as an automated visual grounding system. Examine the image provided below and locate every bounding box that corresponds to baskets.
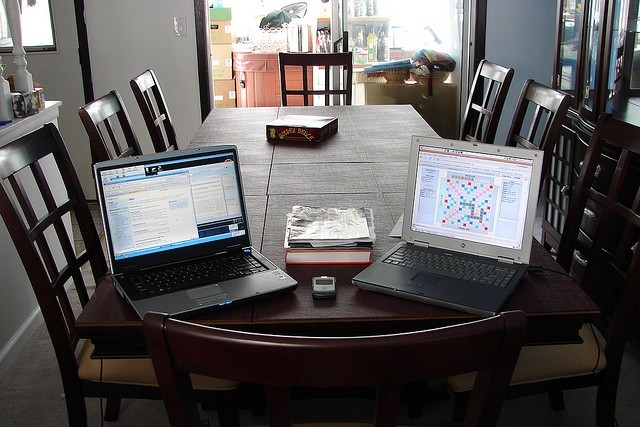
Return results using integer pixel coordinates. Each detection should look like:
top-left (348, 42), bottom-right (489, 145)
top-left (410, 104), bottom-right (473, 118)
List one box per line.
top-left (383, 69), bottom-right (407, 83)
top-left (357, 71), bottom-right (383, 83)
top-left (410, 69), bottom-right (451, 83)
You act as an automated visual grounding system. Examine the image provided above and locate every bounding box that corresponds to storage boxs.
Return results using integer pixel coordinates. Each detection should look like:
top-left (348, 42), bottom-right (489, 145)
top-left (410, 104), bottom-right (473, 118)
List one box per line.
top-left (211, 44), bottom-right (233, 80)
top-left (214, 80), bottom-right (237, 108)
top-left (209, 7), bottom-right (237, 44)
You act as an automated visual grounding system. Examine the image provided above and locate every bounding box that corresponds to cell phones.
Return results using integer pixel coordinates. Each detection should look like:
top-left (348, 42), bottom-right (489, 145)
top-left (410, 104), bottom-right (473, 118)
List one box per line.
top-left (311, 275), bottom-right (337, 301)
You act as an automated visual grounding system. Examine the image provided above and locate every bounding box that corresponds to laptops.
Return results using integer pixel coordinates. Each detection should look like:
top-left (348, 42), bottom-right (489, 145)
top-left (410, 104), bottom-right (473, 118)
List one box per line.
top-left (91, 144), bottom-right (299, 325)
top-left (350, 134), bottom-right (543, 317)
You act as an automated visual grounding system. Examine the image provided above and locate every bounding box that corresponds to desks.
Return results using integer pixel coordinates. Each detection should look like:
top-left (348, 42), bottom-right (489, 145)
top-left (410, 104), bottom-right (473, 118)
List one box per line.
top-left (72, 104), bottom-right (601, 363)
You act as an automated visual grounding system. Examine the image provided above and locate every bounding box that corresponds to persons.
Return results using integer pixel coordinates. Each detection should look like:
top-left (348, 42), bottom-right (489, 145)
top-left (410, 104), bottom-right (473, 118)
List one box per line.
top-left (422, 27), bottom-right (441, 48)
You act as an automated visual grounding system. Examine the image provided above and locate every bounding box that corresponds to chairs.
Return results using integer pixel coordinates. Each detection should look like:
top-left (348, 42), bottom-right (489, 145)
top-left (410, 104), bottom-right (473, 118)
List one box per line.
top-left (130, 69), bottom-right (181, 153)
top-left (78, 90), bottom-right (143, 161)
top-left (279, 52), bottom-right (353, 106)
top-left (504, 78), bottom-right (575, 207)
top-left (428, 112), bottom-right (640, 427)
top-left (0, 122), bottom-right (239, 427)
top-left (142, 309), bottom-right (527, 427)
top-left (459, 59), bottom-right (514, 144)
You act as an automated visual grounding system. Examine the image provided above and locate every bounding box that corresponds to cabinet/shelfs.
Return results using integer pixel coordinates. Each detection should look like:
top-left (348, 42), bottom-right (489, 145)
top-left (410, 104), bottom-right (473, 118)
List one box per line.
top-left (0, 100), bottom-right (81, 374)
top-left (317, 16), bottom-right (389, 69)
top-left (356, 82), bottom-right (456, 140)
top-left (540, 0), bottom-right (640, 342)
top-left (233, 51), bottom-right (313, 108)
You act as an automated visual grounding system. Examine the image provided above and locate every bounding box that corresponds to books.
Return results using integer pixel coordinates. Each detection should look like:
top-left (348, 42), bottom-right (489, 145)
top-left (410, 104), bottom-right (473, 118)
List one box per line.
top-left (284, 205), bottom-right (375, 264)
